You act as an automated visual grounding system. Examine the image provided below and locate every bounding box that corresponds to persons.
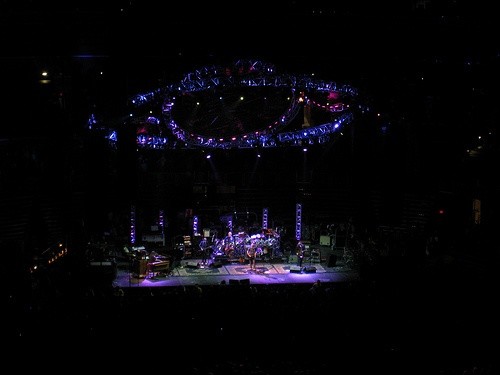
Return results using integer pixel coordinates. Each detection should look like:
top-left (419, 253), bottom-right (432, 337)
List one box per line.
top-left (224, 232), bottom-right (234, 244)
top-left (247, 243), bottom-right (256, 270)
top-left (295, 242), bottom-right (304, 266)
top-left (199, 238), bottom-right (210, 263)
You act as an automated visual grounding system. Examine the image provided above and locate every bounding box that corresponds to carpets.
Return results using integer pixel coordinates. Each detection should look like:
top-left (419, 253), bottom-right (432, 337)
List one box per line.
top-left (174, 264), bottom-right (229, 275)
top-left (225, 264), bottom-right (278, 275)
top-left (273, 263), bottom-right (327, 274)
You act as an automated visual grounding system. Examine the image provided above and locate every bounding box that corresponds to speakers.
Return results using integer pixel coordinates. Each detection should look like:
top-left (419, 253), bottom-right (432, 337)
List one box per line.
top-left (320, 235), bottom-right (330, 246)
top-left (303, 266), bottom-right (316, 274)
top-left (187, 262), bottom-right (200, 269)
top-left (326, 254), bottom-right (336, 267)
top-left (290, 266), bottom-right (301, 274)
